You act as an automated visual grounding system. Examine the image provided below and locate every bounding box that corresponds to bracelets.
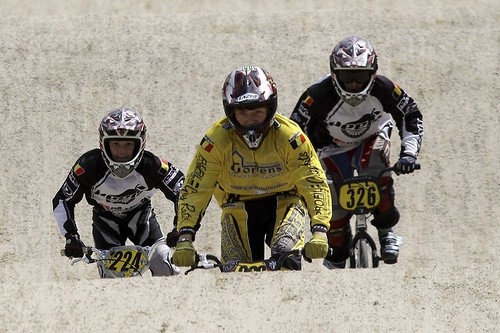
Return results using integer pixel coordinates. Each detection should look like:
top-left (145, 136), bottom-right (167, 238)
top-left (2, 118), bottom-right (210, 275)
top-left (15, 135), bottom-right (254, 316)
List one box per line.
top-left (176, 238), bottom-right (192, 244)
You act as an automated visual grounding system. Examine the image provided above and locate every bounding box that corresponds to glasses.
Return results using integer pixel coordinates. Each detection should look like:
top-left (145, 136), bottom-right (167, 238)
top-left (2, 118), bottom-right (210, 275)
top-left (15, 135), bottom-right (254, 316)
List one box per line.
top-left (337, 68), bottom-right (370, 84)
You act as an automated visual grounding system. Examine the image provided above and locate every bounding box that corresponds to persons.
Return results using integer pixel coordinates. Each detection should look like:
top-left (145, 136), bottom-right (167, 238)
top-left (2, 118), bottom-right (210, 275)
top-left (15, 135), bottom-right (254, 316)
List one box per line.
top-left (173, 66), bottom-right (333, 272)
top-left (289, 36), bottom-right (423, 269)
top-left (52, 108), bottom-right (186, 279)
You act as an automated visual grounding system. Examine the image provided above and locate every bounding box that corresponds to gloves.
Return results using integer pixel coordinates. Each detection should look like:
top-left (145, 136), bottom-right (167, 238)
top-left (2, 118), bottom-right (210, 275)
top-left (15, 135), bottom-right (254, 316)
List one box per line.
top-left (395, 156), bottom-right (416, 175)
top-left (172, 241), bottom-right (195, 266)
top-left (305, 232), bottom-right (328, 259)
top-left (166, 229), bottom-right (180, 247)
top-left (64, 233), bottom-right (86, 258)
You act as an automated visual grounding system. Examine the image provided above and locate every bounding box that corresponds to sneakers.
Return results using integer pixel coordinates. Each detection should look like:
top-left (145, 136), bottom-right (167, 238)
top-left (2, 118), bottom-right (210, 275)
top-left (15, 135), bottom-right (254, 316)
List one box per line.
top-left (377, 229), bottom-right (399, 261)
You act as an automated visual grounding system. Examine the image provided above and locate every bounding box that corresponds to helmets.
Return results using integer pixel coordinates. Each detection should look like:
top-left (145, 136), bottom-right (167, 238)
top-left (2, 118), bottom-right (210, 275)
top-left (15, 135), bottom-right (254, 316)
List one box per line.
top-left (100, 108), bottom-right (147, 178)
top-left (330, 37), bottom-right (379, 106)
top-left (222, 65), bottom-right (278, 148)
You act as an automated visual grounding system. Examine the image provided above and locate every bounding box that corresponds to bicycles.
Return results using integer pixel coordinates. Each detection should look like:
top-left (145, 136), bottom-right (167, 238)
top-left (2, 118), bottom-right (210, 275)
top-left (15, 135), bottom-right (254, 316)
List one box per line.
top-left (323, 163), bottom-right (423, 268)
top-left (167, 247), bottom-right (334, 276)
top-left (59, 245), bottom-right (153, 279)
